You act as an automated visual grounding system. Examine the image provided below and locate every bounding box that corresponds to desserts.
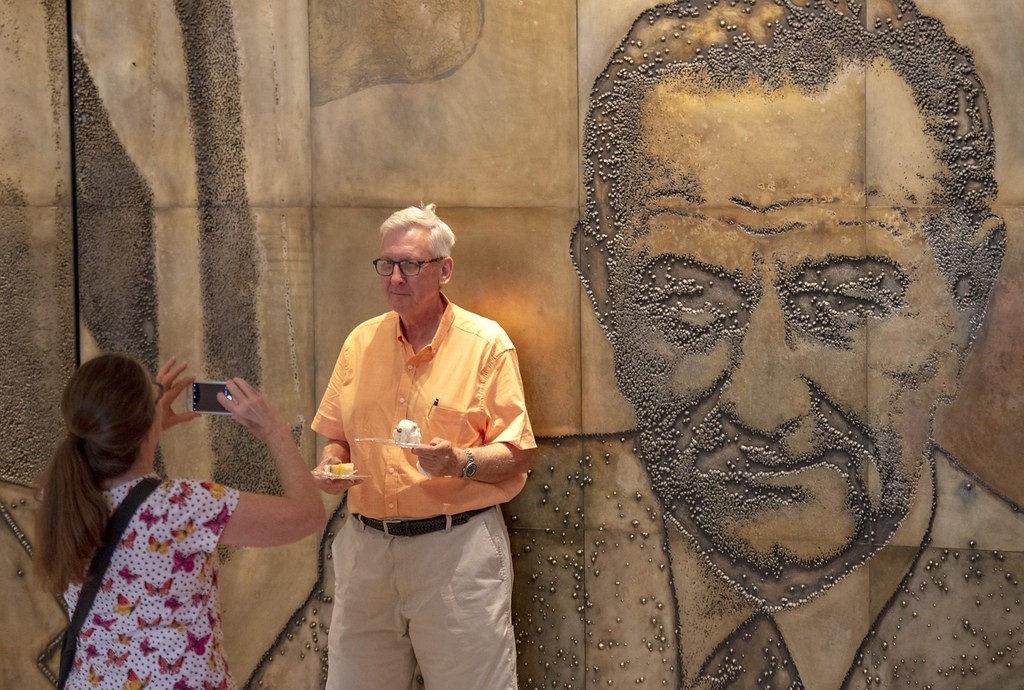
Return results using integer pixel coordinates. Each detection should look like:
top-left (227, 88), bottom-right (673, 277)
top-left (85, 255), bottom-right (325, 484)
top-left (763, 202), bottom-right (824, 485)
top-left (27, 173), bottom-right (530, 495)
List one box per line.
top-left (394, 419), bottom-right (422, 445)
top-left (323, 463), bottom-right (355, 474)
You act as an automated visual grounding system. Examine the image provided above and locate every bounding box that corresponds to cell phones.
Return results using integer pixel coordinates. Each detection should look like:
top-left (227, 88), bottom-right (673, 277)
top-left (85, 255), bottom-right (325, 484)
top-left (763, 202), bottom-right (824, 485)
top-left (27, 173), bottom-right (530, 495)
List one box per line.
top-left (186, 382), bottom-right (238, 416)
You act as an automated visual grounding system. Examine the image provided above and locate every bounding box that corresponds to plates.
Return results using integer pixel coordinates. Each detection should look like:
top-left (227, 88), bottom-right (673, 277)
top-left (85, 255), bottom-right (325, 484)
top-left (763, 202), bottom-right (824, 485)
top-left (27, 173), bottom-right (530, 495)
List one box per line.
top-left (355, 437), bottom-right (433, 449)
top-left (310, 469), bottom-right (374, 481)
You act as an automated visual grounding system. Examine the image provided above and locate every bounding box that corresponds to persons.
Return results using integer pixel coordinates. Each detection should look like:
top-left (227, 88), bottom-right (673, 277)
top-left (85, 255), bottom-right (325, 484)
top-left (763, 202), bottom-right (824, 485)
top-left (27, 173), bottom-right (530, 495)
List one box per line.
top-left (310, 207), bottom-right (537, 690)
top-left (32, 354), bottom-right (328, 690)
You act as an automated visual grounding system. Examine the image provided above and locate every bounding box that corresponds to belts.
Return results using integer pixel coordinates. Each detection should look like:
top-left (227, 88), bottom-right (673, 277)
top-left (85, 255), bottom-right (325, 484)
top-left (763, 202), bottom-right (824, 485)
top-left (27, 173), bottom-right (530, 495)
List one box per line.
top-left (352, 506), bottom-right (495, 537)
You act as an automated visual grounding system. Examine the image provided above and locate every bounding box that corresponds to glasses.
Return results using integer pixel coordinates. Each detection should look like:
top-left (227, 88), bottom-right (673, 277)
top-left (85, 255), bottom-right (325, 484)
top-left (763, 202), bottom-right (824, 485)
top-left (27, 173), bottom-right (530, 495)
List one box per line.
top-left (151, 382), bottom-right (164, 406)
top-left (372, 256), bottom-right (446, 276)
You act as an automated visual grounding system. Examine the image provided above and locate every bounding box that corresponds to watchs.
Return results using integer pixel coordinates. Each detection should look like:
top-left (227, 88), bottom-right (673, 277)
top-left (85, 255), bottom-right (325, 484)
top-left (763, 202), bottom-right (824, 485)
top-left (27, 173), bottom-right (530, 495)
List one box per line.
top-left (461, 448), bottom-right (477, 479)
